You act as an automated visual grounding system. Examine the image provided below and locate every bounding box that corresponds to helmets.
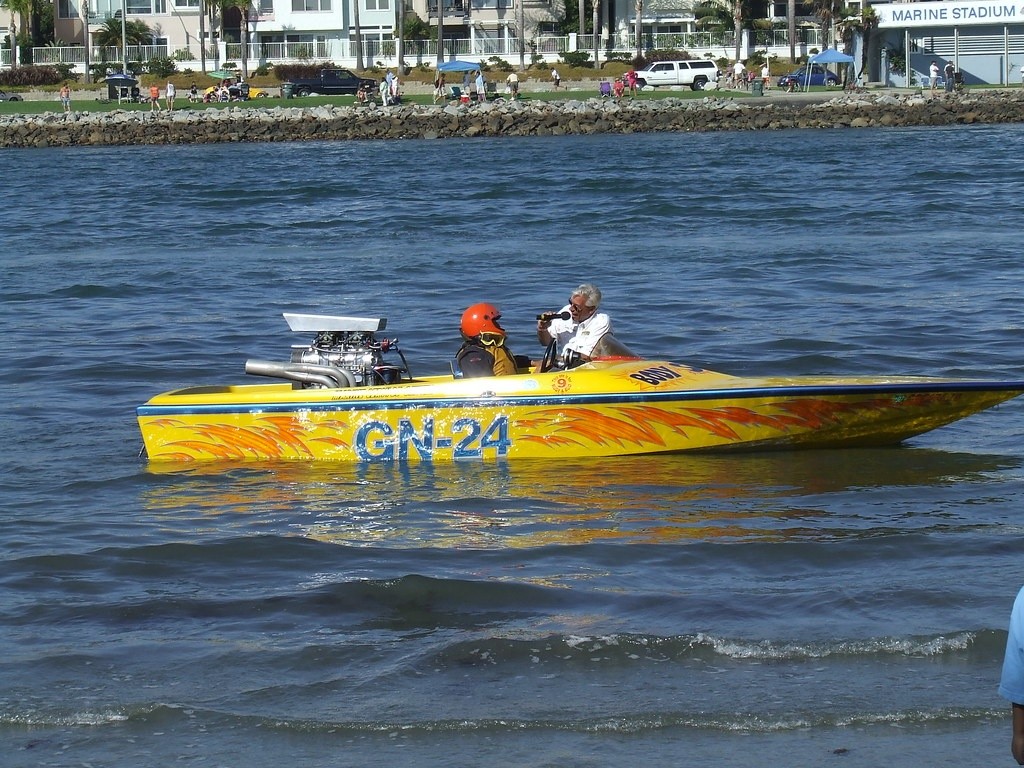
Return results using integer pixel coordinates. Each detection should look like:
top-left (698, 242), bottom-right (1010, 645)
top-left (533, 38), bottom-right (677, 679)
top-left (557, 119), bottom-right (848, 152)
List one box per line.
top-left (460, 303), bottom-right (506, 339)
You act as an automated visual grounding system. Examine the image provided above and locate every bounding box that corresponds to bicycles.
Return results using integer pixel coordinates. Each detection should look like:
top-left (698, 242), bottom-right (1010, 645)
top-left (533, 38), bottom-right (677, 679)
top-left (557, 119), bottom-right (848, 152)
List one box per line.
top-left (781, 74), bottom-right (803, 93)
top-left (842, 79), bottom-right (869, 94)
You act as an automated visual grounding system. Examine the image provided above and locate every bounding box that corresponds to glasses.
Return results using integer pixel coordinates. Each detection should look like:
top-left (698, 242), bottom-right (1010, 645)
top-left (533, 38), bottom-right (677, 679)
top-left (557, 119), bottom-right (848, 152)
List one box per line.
top-left (480, 332), bottom-right (504, 347)
top-left (568, 296), bottom-right (594, 312)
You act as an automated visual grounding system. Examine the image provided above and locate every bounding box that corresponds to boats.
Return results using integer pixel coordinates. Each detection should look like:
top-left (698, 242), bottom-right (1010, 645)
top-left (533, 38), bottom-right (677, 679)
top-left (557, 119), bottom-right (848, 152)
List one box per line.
top-left (134, 310), bottom-right (1024, 465)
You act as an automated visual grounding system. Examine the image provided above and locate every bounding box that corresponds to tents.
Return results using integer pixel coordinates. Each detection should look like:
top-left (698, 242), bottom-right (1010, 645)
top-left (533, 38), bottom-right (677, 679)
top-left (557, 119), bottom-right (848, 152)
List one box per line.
top-left (803, 49), bottom-right (855, 92)
top-left (435, 60), bottom-right (481, 97)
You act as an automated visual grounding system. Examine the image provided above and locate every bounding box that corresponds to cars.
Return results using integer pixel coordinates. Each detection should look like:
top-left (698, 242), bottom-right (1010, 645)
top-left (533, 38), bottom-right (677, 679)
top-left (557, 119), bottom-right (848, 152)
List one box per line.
top-left (203, 85), bottom-right (269, 99)
top-left (776, 65), bottom-right (841, 87)
top-left (0, 89), bottom-right (24, 101)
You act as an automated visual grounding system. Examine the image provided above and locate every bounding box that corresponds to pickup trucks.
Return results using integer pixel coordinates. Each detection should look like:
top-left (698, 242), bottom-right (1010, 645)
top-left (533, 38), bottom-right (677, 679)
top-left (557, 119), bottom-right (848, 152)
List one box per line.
top-left (290, 68), bottom-right (380, 97)
top-left (621, 59), bottom-right (723, 92)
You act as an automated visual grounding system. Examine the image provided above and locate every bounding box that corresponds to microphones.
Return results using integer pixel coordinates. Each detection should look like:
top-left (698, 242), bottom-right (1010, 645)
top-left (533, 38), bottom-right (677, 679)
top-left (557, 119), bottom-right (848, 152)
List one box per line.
top-left (536, 312), bottom-right (570, 320)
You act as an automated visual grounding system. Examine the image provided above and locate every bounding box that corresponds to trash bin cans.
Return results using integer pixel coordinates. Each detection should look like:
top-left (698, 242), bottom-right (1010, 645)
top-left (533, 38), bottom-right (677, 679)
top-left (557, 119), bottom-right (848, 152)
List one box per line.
top-left (750, 79), bottom-right (765, 97)
top-left (280, 82), bottom-right (294, 100)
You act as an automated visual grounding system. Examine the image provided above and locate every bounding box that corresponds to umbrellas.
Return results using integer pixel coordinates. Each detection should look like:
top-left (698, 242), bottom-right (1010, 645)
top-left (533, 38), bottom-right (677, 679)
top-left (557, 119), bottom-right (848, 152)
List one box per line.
top-left (207, 71), bottom-right (237, 88)
top-left (101, 74), bottom-right (139, 105)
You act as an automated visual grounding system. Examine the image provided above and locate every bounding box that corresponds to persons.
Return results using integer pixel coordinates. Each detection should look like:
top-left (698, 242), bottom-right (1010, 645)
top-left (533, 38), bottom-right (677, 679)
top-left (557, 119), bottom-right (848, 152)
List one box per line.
top-left (536, 283), bottom-right (614, 360)
top-left (464, 69), bottom-right (487, 102)
top-left (727, 60), bottom-right (771, 90)
top-left (998, 586), bottom-right (1024, 768)
top-left (434, 73), bottom-right (447, 104)
top-left (601, 69), bottom-right (638, 97)
top-left (456, 303), bottom-right (563, 377)
top-left (357, 88), bottom-right (367, 100)
top-left (929, 61), bottom-right (939, 89)
top-left (506, 72), bottom-right (519, 101)
top-left (166, 81), bottom-right (176, 111)
top-left (379, 69), bottom-right (401, 107)
top-left (550, 67), bottom-right (568, 91)
top-left (944, 60), bottom-right (955, 92)
top-left (191, 80), bottom-right (232, 103)
top-left (60, 83), bottom-right (71, 112)
top-left (150, 83), bottom-right (162, 112)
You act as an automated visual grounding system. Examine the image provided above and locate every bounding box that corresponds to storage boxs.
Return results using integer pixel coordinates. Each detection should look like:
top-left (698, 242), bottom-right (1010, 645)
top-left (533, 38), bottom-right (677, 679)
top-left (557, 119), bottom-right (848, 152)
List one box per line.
top-left (461, 94), bottom-right (469, 102)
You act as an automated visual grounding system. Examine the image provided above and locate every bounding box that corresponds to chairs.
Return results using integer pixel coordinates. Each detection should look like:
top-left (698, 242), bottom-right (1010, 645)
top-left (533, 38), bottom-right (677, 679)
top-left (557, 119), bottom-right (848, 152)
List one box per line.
top-left (920, 76), bottom-right (946, 91)
top-left (599, 82), bottom-right (612, 99)
top-left (487, 82), bottom-right (499, 100)
top-left (956, 72), bottom-right (965, 90)
top-left (451, 87), bottom-right (461, 100)
top-left (131, 88), bottom-right (140, 103)
top-left (121, 88), bottom-right (129, 103)
